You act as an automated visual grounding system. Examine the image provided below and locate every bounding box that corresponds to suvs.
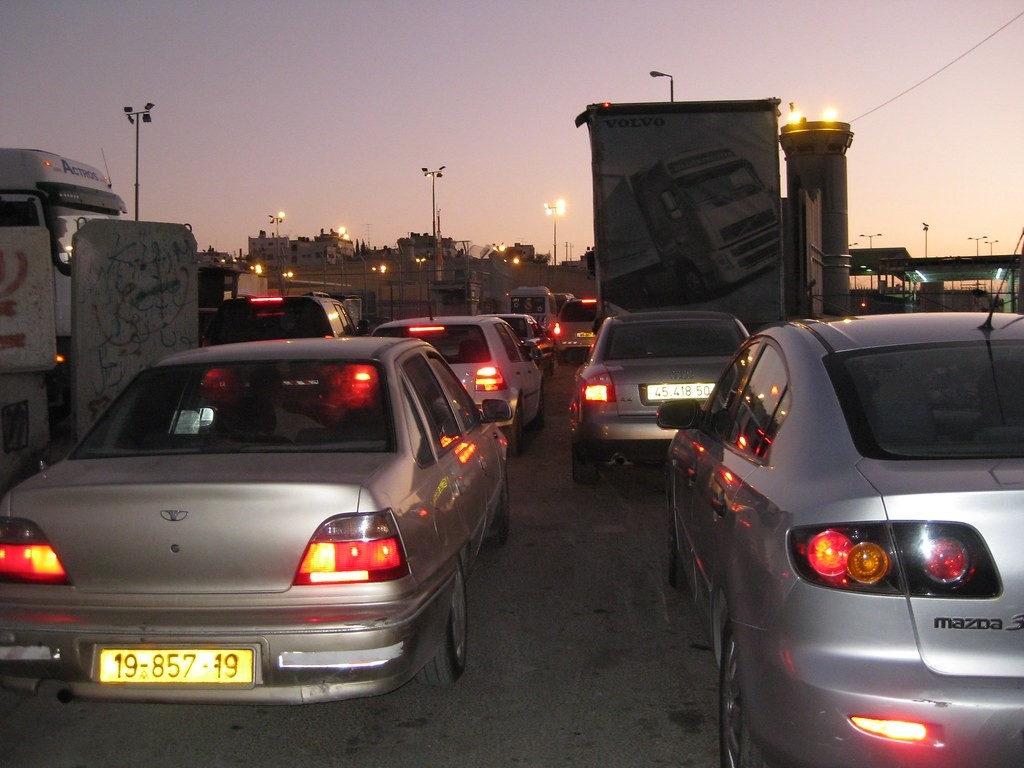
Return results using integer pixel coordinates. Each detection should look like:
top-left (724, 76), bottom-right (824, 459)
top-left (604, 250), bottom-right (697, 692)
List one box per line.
top-left (204, 291), bottom-right (369, 355)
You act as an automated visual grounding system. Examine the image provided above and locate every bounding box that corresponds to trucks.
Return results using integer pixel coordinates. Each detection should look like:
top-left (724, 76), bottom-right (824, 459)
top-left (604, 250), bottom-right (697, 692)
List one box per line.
top-left (0, 148), bottom-right (127, 425)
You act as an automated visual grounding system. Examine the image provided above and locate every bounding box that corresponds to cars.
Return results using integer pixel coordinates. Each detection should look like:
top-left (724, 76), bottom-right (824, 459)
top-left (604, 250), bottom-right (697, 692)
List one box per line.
top-left (476, 285), bottom-right (597, 376)
top-left (656, 311), bottom-right (1024, 768)
top-left (557, 312), bottom-right (751, 486)
top-left (370, 317), bottom-right (546, 459)
top-left (0, 336), bottom-right (513, 706)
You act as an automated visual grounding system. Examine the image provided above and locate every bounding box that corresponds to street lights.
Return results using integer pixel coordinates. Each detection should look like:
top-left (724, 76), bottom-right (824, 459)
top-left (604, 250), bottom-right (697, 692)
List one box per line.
top-left (124, 100), bottom-right (157, 222)
top-left (544, 200), bottom-right (565, 266)
top-left (270, 212), bottom-right (286, 266)
top-left (422, 166), bottom-right (447, 281)
top-left (859, 233), bottom-right (882, 248)
top-left (968, 236), bottom-right (988, 255)
top-left (922, 222), bottom-right (928, 256)
top-left (985, 240), bottom-right (998, 255)
top-left (650, 71), bottom-right (676, 103)
